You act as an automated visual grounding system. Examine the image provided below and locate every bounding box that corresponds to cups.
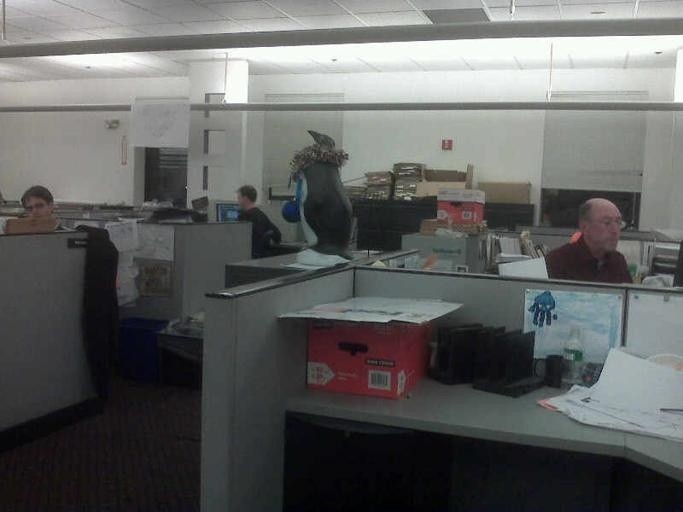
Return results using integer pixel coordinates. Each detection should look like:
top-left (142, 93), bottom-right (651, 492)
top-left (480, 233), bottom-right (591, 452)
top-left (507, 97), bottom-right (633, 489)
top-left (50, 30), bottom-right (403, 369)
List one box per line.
top-left (534, 355), bottom-right (563, 387)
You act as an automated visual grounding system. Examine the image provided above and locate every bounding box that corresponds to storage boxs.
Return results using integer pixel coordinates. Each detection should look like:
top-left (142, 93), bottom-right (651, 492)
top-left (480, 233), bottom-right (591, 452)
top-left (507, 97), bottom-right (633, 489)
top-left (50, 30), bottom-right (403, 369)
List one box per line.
top-left (306, 317), bottom-right (442, 399)
top-left (416, 163), bottom-right (474, 196)
top-left (477, 182), bottom-right (531, 205)
top-left (436, 188), bottom-right (485, 224)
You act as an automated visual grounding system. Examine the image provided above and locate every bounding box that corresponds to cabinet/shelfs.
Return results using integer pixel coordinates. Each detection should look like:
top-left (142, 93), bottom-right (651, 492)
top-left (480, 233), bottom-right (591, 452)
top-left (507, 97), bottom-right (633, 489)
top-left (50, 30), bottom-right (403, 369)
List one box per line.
top-left (351, 199), bottom-right (533, 252)
top-left (539, 187), bottom-right (640, 231)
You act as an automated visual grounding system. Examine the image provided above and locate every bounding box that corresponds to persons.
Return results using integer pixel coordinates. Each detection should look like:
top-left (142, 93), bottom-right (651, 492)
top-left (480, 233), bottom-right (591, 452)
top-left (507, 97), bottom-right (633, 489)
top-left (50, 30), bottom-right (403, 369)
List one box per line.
top-left (545, 197), bottom-right (633, 284)
top-left (234, 185), bottom-right (281, 258)
top-left (19, 185), bottom-right (69, 230)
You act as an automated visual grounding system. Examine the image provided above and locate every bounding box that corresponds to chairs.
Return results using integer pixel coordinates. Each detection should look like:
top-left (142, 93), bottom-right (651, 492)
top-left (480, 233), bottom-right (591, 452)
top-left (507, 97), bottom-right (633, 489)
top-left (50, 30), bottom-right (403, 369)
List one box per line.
top-left (647, 246), bottom-right (679, 275)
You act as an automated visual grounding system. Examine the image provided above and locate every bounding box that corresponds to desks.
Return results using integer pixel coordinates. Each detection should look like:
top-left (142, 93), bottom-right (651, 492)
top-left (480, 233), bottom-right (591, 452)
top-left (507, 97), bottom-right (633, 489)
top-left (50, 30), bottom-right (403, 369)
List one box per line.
top-left (283, 378), bottom-right (683, 512)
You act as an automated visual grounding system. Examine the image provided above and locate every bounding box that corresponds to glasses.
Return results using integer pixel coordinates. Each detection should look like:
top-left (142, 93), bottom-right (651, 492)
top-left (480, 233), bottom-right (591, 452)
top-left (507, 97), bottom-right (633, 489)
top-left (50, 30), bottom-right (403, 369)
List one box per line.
top-left (594, 219), bottom-right (626, 230)
top-left (24, 204), bottom-right (46, 211)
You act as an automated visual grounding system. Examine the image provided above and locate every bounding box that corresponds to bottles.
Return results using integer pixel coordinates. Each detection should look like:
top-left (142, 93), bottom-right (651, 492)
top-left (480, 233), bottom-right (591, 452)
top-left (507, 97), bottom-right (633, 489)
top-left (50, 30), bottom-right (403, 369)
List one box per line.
top-left (561, 329), bottom-right (585, 393)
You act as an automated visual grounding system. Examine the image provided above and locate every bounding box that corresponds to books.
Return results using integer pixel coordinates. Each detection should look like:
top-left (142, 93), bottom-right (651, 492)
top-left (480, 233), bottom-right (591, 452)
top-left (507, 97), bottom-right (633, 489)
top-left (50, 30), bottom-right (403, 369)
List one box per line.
top-left (484, 230), bottom-right (552, 275)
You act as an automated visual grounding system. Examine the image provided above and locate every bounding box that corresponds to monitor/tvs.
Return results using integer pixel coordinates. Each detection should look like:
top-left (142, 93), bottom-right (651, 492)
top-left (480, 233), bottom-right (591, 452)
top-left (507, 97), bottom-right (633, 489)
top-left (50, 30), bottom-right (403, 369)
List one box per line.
top-left (216, 202), bottom-right (240, 223)
top-left (485, 203), bottom-right (535, 230)
